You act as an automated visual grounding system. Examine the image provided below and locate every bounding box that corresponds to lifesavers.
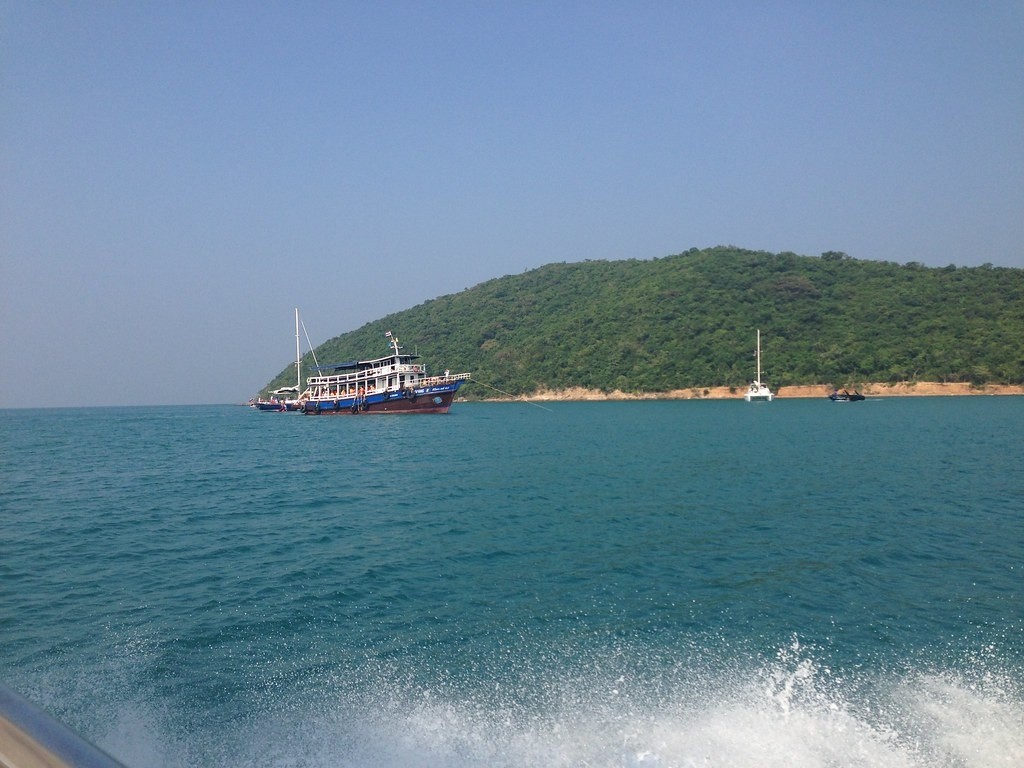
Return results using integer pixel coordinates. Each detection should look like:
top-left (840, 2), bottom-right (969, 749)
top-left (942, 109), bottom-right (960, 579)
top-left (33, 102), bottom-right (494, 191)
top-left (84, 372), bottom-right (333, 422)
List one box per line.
top-left (300, 405), bottom-right (306, 413)
top-left (350, 405), bottom-right (357, 413)
top-left (406, 390), bottom-right (416, 400)
top-left (334, 401), bottom-right (339, 409)
top-left (362, 402), bottom-right (368, 409)
top-left (313, 405), bottom-right (320, 413)
top-left (382, 392), bottom-right (390, 399)
top-left (413, 365), bottom-right (419, 372)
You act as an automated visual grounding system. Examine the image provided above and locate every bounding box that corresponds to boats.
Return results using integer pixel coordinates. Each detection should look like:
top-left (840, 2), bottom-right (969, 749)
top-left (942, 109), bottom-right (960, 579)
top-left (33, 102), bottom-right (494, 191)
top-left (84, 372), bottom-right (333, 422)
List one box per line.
top-left (294, 307), bottom-right (472, 414)
top-left (829, 388), bottom-right (866, 403)
top-left (250, 403), bottom-right (256, 408)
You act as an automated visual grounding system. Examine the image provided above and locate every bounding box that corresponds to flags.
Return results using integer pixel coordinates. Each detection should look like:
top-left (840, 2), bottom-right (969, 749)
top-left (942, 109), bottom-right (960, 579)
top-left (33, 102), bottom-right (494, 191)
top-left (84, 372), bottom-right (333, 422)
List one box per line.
top-left (385, 331), bottom-right (391, 337)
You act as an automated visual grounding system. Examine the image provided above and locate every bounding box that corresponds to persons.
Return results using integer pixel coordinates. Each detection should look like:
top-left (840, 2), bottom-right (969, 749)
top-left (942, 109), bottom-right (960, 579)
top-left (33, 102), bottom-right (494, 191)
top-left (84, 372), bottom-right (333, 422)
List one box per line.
top-left (340, 390), bottom-right (346, 394)
top-left (349, 387), bottom-right (356, 393)
top-left (370, 384), bottom-right (375, 390)
top-left (360, 386), bottom-right (365, 392)
top-left (330, 390), bottom-right (336, 395)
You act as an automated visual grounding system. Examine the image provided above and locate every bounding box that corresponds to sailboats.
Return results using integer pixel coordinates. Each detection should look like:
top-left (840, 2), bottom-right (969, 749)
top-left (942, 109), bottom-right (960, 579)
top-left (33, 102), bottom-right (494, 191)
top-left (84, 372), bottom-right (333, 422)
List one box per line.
top-left (744, 328), bottom-right (775, 403)
top-left (255, 307), bottom-right (300, 412)
